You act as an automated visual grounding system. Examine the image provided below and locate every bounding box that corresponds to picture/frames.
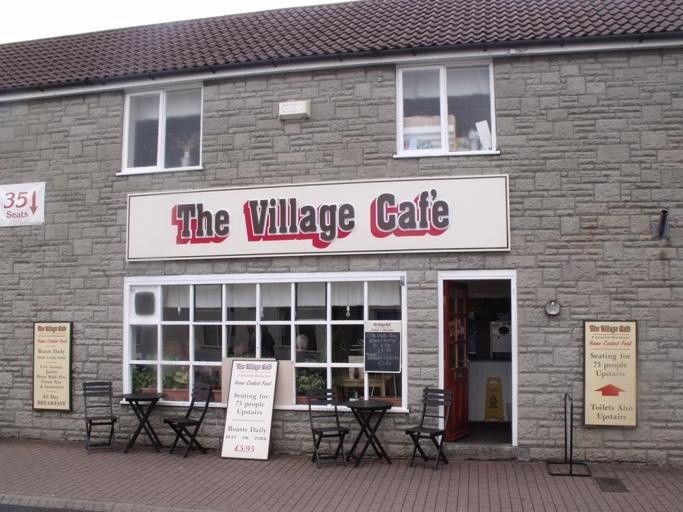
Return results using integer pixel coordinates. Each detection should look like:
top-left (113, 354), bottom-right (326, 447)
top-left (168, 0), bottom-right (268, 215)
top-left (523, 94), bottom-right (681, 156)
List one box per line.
top-left (33, 320), bottom-right (73, 412)
top-left (583, 319), bottom-right (638, 428)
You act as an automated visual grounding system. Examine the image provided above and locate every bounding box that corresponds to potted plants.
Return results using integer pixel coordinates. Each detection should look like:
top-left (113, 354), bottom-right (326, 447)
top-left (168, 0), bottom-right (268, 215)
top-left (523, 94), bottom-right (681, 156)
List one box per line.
top-left (168, 131), bottom-right (199, 166)
top-left (133, 350), bottom-right (327, 404)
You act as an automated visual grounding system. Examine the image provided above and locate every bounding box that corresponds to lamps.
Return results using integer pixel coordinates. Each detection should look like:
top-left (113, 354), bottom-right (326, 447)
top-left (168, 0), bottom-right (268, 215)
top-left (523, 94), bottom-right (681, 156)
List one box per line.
top-left (545, 300), bottom-right (561, 315)
top-left (345, 282), bottom-right (350, 317)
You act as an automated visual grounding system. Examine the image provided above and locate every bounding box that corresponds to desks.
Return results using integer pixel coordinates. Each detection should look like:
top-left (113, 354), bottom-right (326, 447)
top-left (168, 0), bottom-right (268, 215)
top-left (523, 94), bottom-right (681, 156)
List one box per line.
top-left (342, 378), bottom-right (387, 397)
top-left (344, 399), bottom-right (393, 469)
top-left (113, 392), bottom-right (166, 454)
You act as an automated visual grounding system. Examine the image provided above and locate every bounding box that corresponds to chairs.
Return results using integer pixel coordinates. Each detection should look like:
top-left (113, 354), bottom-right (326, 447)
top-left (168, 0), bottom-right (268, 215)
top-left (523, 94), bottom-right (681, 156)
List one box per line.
top-left (405, 386), bottom-right (454, 470)
top-left (164, 383), bottom-right (213, 458)
top-left (305, 386), bottom-right (350, 469)
top-left (82, 379), bottom-right (120, 454)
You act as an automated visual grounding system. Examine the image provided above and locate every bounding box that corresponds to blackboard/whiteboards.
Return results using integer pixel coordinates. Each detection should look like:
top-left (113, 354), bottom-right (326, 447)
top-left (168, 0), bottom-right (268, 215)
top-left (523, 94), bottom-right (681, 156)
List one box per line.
top-left (365, 332), bottom-right (400, 373)
